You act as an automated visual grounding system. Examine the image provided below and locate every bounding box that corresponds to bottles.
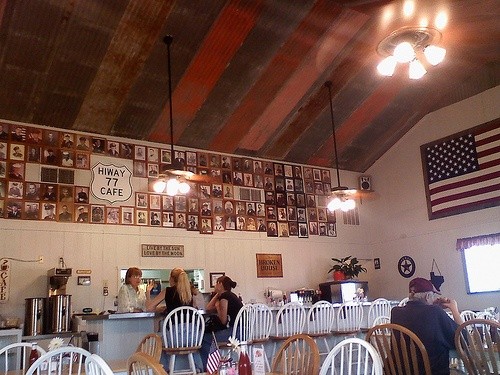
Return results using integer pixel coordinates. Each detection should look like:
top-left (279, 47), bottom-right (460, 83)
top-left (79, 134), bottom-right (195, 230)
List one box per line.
top-left (59, 258), bottom-right (64, 269)
top-left (29, 342), bottom-right (37, 375)
top-left (238, 341), bottom-right (252, 375)
top-left (283, 291), bottom-right (287, 305)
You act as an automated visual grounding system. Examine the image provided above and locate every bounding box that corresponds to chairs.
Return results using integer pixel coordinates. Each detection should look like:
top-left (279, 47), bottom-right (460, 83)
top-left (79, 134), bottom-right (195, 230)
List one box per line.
top-left (0, 299), bottom-right (500, 375)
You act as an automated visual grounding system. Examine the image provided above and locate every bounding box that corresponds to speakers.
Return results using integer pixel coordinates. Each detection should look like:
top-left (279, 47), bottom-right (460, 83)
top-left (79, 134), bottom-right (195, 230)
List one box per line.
top-left (360, 176), bottom-right (370, 190)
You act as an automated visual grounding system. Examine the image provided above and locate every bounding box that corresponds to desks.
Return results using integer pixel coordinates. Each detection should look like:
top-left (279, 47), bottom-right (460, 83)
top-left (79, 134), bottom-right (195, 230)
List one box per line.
top-left (373, 334), bottom-right (500, 361)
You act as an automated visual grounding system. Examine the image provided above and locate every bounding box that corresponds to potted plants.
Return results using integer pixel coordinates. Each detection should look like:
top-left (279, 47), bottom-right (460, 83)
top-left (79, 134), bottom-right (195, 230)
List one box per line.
top-left (328, 256), bottom-right (369, 281)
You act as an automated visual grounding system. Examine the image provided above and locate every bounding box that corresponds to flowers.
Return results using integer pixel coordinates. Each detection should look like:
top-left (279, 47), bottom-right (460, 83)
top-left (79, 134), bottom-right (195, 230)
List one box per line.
top-left (225, 336), bottom-right (239, 361)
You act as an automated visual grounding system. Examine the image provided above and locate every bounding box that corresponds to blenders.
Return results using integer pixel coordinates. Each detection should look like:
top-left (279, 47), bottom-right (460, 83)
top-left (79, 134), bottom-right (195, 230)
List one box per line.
top-left (46, 267), bottom-right (72, 333)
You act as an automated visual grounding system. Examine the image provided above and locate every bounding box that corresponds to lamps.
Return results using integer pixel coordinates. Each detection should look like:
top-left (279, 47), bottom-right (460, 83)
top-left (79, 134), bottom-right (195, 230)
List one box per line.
top-left (323, 81), bottom-right (357, 211)
top-left (377, 26), bottom-right (446, 79)
top-left (154, 37), bottom-right (193, 196)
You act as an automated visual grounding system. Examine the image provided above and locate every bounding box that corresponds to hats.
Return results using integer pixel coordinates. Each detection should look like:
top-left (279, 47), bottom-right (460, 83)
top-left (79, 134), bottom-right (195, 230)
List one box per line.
top-left (409, 277), bottom-right (442, 295)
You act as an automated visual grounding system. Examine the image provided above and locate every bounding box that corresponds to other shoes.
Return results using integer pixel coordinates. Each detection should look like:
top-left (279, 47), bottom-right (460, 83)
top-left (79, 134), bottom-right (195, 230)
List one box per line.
top-left (196, 367), bottom-right (207, 375)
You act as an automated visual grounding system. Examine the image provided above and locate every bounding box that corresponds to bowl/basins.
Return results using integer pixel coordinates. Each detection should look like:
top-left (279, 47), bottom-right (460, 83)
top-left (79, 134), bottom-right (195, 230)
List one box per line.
top-left (0, 318), bottom-right (20, 329)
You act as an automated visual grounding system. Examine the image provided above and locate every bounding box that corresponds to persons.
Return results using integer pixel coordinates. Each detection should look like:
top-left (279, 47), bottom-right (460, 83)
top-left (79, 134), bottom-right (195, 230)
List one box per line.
top-left (149, 268), bottom-right (204, 373)
top-left (0, 125), bottom-right (336, 236)
top-left (391, 278), bottom-right (469, 375)
top-left (196, 276), bottom-right (248, 373)
top-left (116, 267), bottom-right (154, 313)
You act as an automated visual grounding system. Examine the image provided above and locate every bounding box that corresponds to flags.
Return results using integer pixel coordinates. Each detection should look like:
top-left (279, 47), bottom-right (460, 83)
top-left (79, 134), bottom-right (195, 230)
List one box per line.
top-left (424, 123), bottom-right (500, 217)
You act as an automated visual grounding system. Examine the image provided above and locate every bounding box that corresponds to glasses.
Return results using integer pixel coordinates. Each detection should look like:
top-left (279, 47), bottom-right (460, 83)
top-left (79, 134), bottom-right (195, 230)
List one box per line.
top-left (130, 275), bottom-right (142, 279)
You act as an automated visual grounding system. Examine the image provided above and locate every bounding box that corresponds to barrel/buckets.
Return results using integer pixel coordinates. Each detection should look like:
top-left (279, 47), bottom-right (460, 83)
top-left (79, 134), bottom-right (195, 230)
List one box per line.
top-left (51, 295), bottom-right (73, 332)
top-left (23, 297), bottom-right (46, 336)
